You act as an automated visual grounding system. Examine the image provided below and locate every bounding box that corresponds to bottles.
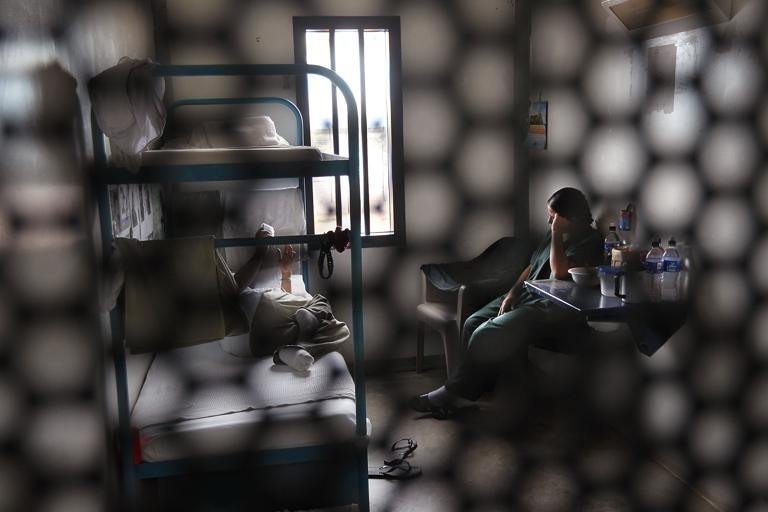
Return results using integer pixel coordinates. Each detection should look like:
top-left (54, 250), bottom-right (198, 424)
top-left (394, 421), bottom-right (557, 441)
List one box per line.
top-left (645, 238), bottom-right (665, 303)
top-left (603, 222), bottom-right (622, 265)
top-left (623, 239), bottom-right (633, 255)
top-left (660, 240), bottom-right (681, 303)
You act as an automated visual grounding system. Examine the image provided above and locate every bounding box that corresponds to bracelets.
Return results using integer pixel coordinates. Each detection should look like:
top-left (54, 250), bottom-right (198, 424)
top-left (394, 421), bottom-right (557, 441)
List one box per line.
top-left (281, 277), bottom-right (290, 282)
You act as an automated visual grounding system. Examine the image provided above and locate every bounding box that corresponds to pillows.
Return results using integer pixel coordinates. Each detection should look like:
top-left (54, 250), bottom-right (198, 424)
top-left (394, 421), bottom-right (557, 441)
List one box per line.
top-left (189, 115), bottom-right (280, 149)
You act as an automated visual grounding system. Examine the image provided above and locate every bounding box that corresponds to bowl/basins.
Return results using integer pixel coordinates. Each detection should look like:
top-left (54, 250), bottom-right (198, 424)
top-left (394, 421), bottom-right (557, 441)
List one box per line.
top-left (567, 266), bottom-right (600, 287)
top-left (598, 265), bottom-right (623, 298)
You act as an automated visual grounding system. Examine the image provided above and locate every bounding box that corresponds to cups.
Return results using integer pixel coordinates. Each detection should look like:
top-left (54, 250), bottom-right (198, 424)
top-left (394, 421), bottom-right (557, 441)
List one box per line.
top-left (614, 266), bottom-right (653, 305)
top-left (617, 209), bottom-right (633, 231)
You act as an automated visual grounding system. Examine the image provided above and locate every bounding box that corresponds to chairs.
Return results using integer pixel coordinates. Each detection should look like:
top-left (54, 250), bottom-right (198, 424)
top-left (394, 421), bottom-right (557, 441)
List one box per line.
top-left (416, 237), bottom-right (538, 378)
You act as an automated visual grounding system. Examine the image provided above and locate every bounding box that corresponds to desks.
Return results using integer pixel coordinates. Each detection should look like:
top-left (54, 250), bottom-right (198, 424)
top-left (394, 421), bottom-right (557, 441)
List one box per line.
top-left (523, 279), bottom-right (692, 357)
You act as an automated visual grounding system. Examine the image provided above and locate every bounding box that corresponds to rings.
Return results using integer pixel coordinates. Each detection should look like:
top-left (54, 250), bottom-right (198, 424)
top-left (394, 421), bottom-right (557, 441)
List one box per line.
top-left (283, 253), bottom-right (287, 256)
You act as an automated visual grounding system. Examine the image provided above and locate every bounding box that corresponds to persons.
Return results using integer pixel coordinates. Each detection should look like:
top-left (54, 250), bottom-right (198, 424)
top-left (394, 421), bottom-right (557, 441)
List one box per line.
top-left (234, 222), bottom-right (349, 372)
top-left (411, 185), bottom-right (603, 420)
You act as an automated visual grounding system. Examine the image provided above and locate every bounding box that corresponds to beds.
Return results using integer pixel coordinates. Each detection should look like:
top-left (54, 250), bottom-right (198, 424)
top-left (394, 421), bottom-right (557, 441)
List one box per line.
top-left (91, 64), bottom-right (368, 512)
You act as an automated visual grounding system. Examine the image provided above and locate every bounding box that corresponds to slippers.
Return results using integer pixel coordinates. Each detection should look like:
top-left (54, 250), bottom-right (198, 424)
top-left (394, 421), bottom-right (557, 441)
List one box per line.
top-left (368, 459), bottom-right (422, 480)
top-left (410, 393), bottom-right (441, 412)
top-left (384, 438), bottom-right (417, 465)
top-left (432, 405), bottom-right (479, 419)
